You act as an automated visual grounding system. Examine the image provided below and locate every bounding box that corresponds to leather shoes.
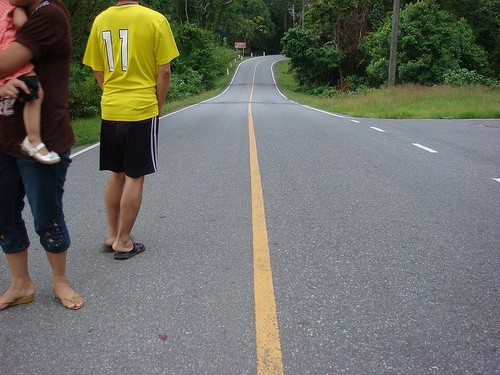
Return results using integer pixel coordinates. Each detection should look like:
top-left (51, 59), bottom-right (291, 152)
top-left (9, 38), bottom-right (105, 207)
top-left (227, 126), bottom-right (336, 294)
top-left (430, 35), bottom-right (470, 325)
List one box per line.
top-left (20, 137), bottom-right (60, 164)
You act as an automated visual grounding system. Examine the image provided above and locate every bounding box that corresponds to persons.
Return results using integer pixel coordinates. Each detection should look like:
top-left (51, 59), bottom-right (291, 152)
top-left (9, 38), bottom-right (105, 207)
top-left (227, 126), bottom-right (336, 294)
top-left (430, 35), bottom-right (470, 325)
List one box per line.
top-left (0, 0), bottom-right (84, 310)
top-left (82, 0), bottom-right (179, 258)
top-left (0, 0), bottom-right (61, 164)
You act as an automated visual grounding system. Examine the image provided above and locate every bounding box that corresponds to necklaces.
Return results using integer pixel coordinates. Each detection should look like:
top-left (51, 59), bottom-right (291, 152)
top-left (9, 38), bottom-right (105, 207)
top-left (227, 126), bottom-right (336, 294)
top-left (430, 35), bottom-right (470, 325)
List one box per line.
top-left (115, 2), bottom-right (138, 6)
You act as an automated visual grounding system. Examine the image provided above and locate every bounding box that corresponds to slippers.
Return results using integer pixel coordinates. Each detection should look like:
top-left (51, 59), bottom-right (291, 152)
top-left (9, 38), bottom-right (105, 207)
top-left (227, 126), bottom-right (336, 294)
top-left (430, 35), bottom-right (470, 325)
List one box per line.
top-left (113, 243), bottom-right (145, 260)
top-left (0, 295), bottom-right (35, 311)
top-left (54, 284), bottom-right (84, 310)
top-left (103, 244), bottom-right (115, 252)
